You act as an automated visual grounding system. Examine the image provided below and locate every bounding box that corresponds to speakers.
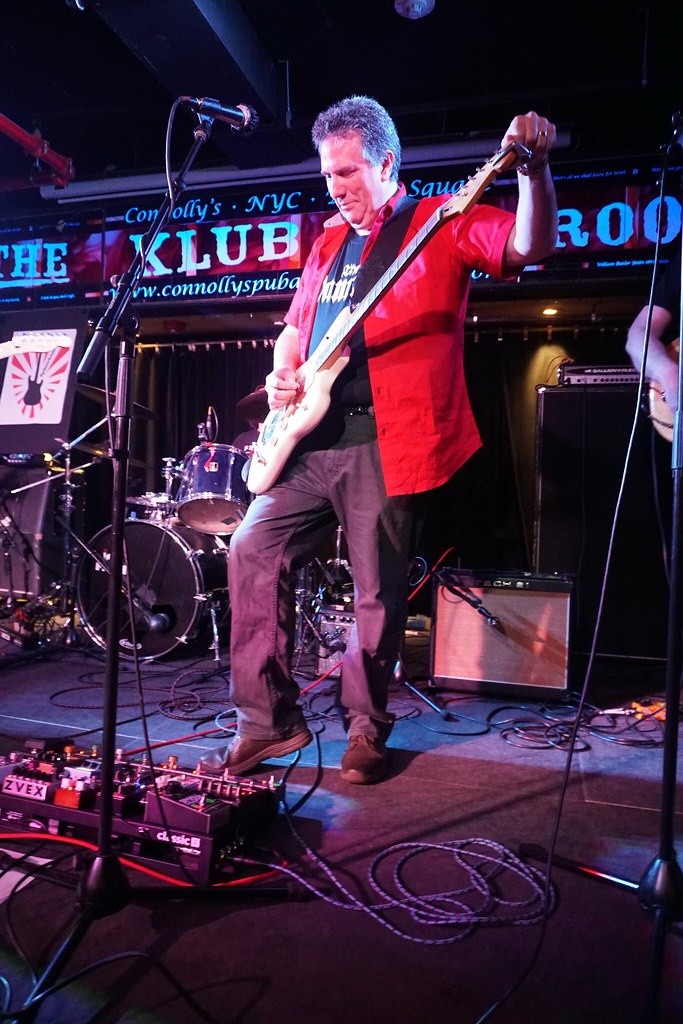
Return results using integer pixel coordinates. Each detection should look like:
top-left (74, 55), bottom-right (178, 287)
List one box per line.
top-left (429, 572), bottom-right (574, 702)
top-left (314, 611), bottom-right (355, 678)
top-left (530, 384), bottom-right (683, 668)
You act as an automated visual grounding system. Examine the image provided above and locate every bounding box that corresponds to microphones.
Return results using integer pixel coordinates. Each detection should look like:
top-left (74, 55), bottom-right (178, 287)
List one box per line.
top-left (205, 407), bottom-right (213, 440)
top-left (145, 613), bottom-right (172, 633)
top-left (325, 640), bottom-right (344, 660)
top-left (179, 96), bottom-right (260, 135)
top-left (488, 616), bottom-right (500, 628)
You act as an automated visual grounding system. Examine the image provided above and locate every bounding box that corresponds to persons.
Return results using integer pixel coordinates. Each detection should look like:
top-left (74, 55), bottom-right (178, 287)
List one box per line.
top-left (232, 385), bottom-right (268, 452)
top-left (196, 95), bottom-right (557, 786)
top-left (626, 252), bottom-right (683, 415)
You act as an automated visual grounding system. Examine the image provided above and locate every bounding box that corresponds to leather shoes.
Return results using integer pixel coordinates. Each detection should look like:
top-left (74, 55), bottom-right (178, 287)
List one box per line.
top-left (340, 733), bottom-right (389, 783)
top-left (200, 724), bottom-right (313, 776)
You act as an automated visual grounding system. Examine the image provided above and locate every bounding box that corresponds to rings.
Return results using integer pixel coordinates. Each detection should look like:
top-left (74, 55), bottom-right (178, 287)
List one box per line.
top-left (539, 131), bottom-right (548, 136)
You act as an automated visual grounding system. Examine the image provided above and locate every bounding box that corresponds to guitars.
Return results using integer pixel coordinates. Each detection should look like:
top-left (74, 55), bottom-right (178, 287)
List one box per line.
top-left (242, 139), bottom-right (521, 497)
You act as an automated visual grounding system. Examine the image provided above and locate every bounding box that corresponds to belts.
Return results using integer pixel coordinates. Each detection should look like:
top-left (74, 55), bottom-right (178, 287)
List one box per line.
top-left (329, 405), bottom-right (375, 416)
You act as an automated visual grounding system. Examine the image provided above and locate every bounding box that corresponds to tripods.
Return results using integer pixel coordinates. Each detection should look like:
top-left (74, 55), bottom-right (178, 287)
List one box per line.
top-left (1, 110), bottom-right (303, 1024)
top-left (291, 526), bottom-right (450, 722)
top-left (0, 404), bottom-right (130, 672)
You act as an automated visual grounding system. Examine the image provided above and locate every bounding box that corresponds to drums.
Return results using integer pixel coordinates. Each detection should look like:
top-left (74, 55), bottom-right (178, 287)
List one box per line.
top-left (177, 443), bottom-right (255, 536)
top-left (123, 496), bottom-right (178, 525)
top-left (75, 520), bottom-right (232, 661)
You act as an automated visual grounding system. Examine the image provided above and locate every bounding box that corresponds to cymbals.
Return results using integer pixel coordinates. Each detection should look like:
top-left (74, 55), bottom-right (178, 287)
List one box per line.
top-left (73, 382), bottom-right (155, 417)
top-left (57, 438), bottom-right (154, 472)
top-left (237, 386), bottom-right (268, 424)
